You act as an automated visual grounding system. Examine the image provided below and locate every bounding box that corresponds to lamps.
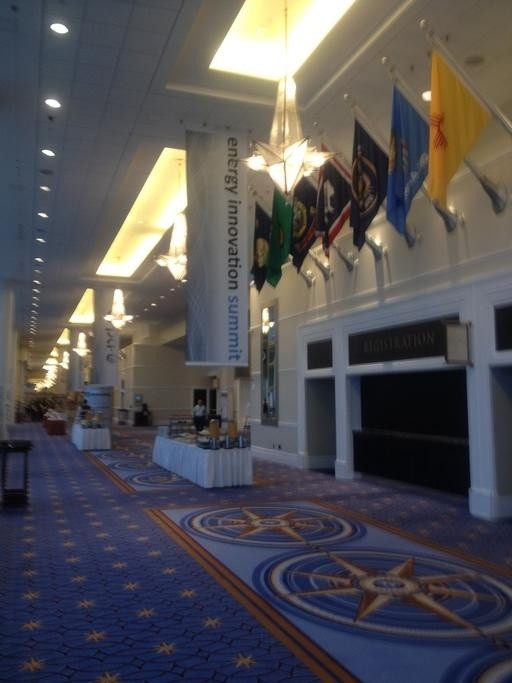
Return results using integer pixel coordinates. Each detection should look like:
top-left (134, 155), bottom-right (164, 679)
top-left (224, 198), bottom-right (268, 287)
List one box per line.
top-left (240, 0), bottom-right (338, 197)
top-left (58, 331), bottom-right (93, 371)
top-left (152, 158), bottom-right (188, 282)
top-left (103, 257), bottom-right (135, 330)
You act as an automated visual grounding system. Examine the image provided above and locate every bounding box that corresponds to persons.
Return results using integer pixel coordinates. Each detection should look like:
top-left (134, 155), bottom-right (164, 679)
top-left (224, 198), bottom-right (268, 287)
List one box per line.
top-left (191, 399), bottom-right (206, 432)
top-left (78, 399), bottom-right (92, 418)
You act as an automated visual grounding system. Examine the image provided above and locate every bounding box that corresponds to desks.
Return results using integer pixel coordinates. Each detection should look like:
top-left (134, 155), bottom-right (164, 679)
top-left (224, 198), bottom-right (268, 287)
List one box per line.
top-left (43, 414), bottom-right (65, 436)
top-left (71, 421), bottom-right (111, 452)
top-left (153, 432), bottom-right (251, 489)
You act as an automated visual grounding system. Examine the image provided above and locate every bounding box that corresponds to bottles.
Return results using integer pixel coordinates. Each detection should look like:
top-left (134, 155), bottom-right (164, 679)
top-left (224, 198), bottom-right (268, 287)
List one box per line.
top-left (227, 420), bottom-right (237, 436)
top-left (209, 419), bottom-right (221, 437)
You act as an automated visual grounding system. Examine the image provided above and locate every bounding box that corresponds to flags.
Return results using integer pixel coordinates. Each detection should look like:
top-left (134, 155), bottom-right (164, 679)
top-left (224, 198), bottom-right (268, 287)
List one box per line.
top-left (386, 86), bottom-right (430, 246)
top-left (426, 48), bottom-right (493, 209)
top-left (290, 175), bottom-right (317, 272)
top-left (348, 114), bottom-right (389, 251)
top-left (263, 188), bottom-right (294, 287)
top-left (312, 144), bottom-right (352, 259)
top-left (251, 199), bottom-right (271, 294)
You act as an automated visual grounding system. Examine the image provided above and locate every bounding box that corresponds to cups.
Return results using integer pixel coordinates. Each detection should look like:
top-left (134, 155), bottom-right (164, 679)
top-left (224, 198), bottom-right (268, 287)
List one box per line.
top-left (224, 436), bottom-right (234, 450)
top-left (209, 437), bottom-right (222, 450)
top-left (238, 434), bottom-right (249, 447)
